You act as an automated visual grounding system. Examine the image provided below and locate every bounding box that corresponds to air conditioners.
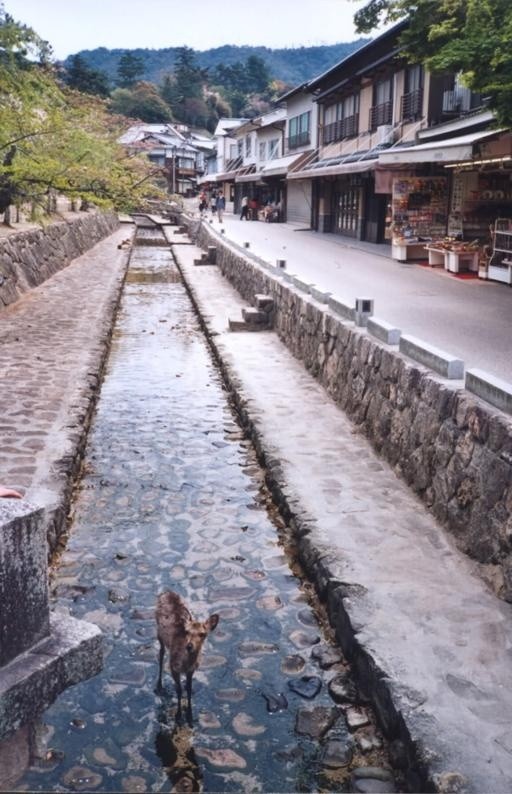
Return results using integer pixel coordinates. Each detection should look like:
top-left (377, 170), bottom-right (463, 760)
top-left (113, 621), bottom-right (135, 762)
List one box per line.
top-left (442, 90), bottom-right (458, 113)
top-left (375, 125), bottom-right (396, 147)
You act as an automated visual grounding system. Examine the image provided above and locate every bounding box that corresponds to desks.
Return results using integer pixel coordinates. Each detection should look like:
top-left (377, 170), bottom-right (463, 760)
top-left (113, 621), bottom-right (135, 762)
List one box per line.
top-left (392, 240), bottom-right (439, 263)
top-left (423, 246), bottom-right (480, 274)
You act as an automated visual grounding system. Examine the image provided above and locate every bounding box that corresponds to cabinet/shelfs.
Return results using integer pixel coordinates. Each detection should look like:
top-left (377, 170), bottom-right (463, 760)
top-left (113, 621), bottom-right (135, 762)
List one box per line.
top-left (453, 197), bottom-right (512, 244)
top-left (488, 218), bottom-right (512, 286)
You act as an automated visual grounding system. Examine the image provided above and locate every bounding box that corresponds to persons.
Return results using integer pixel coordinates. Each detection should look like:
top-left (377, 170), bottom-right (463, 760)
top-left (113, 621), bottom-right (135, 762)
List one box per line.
top-left (240, 194), bottom-right (249, 221)
top-left (209, 193), bottom-right (217, 216)
top-left (214, 190), bottom-right (224, 223)
top-left (249, 198), bottom-right (258, 220)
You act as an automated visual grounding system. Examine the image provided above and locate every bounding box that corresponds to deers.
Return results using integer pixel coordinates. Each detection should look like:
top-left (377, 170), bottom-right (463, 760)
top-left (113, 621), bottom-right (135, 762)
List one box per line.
top-left (154, 590), bottom-right (219, 717)
